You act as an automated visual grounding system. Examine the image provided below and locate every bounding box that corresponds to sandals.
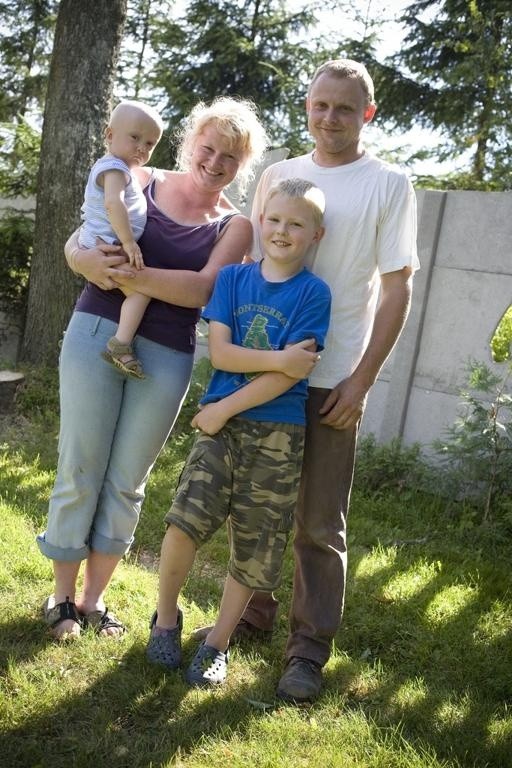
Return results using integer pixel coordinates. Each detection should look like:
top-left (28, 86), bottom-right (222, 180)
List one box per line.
top-left (100, 334), bottom-right (147, 381)
top-left (187, 636), bottom-right (230, 691)
top-left (144, 605), bottom-right (184, 674)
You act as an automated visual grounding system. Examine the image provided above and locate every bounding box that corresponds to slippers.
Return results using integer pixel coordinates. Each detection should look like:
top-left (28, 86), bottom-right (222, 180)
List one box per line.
top-left (45, 591), bottom-right (81, 645)
top-left (78, 606), bottom-right (127, 643)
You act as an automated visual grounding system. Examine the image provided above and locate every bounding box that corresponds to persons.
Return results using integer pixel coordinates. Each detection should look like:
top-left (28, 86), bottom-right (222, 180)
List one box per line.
top-left (78, 100), bottom-right (164, 381)
top-left (36, 96), bottom-right (272, 643)
top-left (191, 59), bottom-right (420, 704)
top-left (145, 177), bottom-right (332, 687)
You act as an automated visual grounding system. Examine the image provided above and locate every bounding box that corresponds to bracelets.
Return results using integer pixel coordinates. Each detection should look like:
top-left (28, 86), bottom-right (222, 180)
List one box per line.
top-left (69, 247), bottom-right (80, 275)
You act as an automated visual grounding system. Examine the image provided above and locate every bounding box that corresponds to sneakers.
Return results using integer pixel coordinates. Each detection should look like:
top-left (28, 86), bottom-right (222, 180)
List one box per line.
top-left (277, 656), bottom-right (322, 704)
top-left (191, 615), bottom-right (273, 646)
